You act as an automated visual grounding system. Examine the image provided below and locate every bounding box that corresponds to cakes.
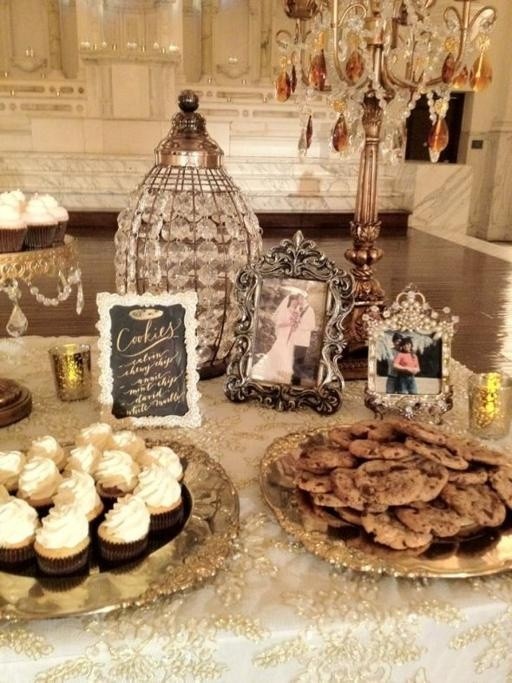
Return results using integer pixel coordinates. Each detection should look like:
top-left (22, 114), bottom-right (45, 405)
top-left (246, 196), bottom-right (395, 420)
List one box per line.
top-left (0, 189), bottom-right (70, 253)
top-left (0, 423), bottom-right (185, 578)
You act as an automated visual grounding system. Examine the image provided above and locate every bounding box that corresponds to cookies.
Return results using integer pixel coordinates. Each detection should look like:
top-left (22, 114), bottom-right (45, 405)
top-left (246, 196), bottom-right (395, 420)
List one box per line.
top-left (294, 416), bottom-right (512, 560)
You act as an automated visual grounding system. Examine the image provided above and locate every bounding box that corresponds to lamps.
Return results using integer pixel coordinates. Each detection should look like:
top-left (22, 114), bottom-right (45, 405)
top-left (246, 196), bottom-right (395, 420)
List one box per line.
top-left (270, 0), bottom-right (498, 383)
top-left (113, 85), bottom-right (264, 383)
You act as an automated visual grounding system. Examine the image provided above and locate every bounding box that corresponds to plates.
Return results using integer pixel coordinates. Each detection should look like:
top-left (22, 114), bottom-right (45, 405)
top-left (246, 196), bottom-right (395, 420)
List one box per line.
top-left (0, 436), bottom-right (240, 623)
top-left (260, 419), bottom-right (512, 579)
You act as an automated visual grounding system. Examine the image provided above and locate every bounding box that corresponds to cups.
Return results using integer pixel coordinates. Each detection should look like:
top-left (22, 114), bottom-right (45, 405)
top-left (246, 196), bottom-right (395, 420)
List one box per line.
top-left (48, 343), bottom-right (92, 402)
top-left (467, 371), bottom-right (512, 441)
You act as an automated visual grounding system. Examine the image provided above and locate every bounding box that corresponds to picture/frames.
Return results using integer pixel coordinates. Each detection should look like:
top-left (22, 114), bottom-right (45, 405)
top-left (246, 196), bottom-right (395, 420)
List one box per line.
top-left (222, 227), bottom-right (358, 418)
top-left (94, 288), bottom-right (205, 432)
top-left (360, 281), bottom-right (461, 426)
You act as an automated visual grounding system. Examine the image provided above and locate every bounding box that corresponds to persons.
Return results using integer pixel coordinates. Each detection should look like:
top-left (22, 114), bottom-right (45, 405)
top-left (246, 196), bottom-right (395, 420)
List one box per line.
top-left (251, 294), bottom-right (298, 383)
top-left (394, 338), bottom-right (422, 394)
top-left (386, 334), bottom-right (404, 392)
top-left (290, 288), bottom-right (316, 385)
